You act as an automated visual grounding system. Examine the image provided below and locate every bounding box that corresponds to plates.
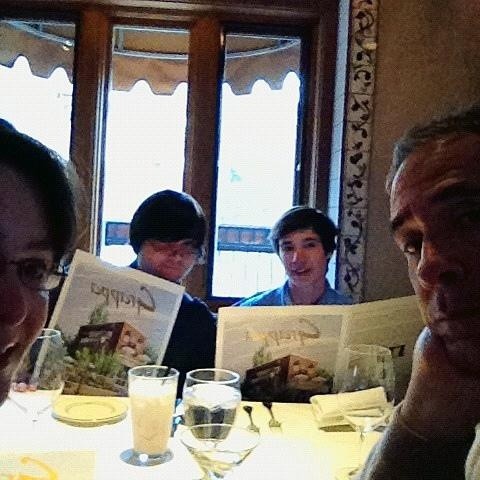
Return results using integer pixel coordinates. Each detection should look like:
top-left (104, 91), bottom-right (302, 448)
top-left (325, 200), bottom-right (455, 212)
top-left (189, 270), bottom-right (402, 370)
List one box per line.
top-left (50, 396), bottom-right (127, 424)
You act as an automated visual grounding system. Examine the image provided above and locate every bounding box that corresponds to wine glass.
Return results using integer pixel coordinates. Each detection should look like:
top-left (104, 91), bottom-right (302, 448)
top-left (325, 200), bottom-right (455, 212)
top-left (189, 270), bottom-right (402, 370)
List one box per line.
top-left (334, 344), bottom-right (396, 477)
top-left (5, 329), bottom-right (64, 474)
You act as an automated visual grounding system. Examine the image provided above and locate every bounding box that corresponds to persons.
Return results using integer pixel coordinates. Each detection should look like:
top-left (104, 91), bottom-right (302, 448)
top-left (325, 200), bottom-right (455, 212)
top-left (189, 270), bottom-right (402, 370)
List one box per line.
top-left (230, 203), bottom-right (358, 306)
top-left (361, 106), bottom-right (478, 480)
top-left (0, 119), bottom-right (78, 413)
top-left (125, 187), bottom-right (218, 396)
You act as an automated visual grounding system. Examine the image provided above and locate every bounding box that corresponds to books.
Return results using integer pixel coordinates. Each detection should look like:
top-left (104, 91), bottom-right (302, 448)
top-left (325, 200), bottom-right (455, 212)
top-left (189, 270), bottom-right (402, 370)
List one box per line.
top-left (215, 292), bottom-right (438, 402)
top-left (33, 247), bottom-right (186, 396)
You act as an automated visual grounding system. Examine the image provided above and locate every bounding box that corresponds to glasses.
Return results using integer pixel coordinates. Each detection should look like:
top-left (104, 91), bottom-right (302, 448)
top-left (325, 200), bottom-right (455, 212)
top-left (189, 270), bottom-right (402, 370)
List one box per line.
top-left (2, 257), bottom-right (69, 291)
top-left (149, 242), bottom-right (202, 260)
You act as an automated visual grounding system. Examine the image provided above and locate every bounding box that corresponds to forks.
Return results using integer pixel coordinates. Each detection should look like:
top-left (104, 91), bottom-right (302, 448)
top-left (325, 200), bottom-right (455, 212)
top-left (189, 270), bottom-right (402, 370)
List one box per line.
top-left (263, 400), bottom-right (282, 433)
top-left (244, 404), bottom-right (262, 435)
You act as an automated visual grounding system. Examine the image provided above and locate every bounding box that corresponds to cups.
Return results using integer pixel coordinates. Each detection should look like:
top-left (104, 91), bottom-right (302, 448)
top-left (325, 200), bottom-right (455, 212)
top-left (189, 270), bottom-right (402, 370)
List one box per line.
top-left (126, 364), bottom-right (260, 479)
